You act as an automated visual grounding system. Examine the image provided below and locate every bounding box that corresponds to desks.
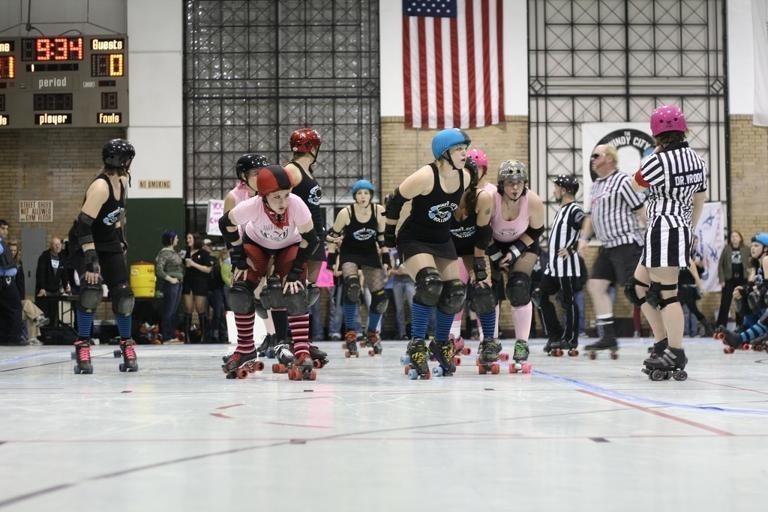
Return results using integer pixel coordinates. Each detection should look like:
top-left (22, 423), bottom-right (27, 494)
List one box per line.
top-left (35, 295), bottom-right (80, 345)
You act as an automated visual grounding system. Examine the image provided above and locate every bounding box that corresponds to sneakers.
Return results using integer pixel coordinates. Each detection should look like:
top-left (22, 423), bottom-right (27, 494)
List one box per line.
top-left (163, 338), bottom-right (184, 345)
top-left (35, 316), bottom-right (49, 328)
top-left (25, 338), bottom-right (43, 345)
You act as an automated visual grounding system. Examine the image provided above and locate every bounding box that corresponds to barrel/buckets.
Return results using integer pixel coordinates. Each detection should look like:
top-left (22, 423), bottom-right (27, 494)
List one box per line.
top-left (129, 260), bottom-right (157, 299)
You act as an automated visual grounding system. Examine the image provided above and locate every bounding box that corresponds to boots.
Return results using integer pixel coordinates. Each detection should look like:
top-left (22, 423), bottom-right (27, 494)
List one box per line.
top-left (183, 312), bottom-right (193, 344)
top-left (699, 316), bottom-right (713, 337)
top-left (198, 311), bottom-right (210, 344)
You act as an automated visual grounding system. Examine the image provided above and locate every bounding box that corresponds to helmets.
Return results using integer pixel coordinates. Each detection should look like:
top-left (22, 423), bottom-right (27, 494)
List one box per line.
top-left (290, 129), bottom-right (322, 153)
top-left (351, 179), bottom-right (375, 202)
top-left (431, 128), bottom-right (528, 190)
top-left (650, 105), bottom-right (687, 137)
top-left (102, 138), bottom-right (136, 169)
top-left (552, 174), bottom-right (579, 196)
top-left (236, 152), bottom-right (270, 184)
top-left (256, 165), bottom-right (295, 197)
top-left (751, 232), bottom-right (767, 248)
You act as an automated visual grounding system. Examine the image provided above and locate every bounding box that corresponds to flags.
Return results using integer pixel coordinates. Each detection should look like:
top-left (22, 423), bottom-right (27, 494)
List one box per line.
top-left (402, 1), bottom-right (505, 128)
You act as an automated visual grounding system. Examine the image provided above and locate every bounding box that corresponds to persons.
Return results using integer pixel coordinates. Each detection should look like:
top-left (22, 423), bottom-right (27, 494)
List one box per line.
top-left (152, 229), bottom-right (233, 344)
top-left (681, 230), bottom-right (767, 354)
top-left (68, 138), bottom-right (139, 375)
top-left (624, 106), bottom-right (708, 371)
top-left (218, 129), bottom-right (650, 380)
top-left (0, 219), bottom-right (81, 345)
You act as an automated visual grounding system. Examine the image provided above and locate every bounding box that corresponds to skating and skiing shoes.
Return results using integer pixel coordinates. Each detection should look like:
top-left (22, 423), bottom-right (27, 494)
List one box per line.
top-left (712, 324), bottom-right (767, 354)
top-left (360, 329), bottom-right (382, 356)
top-left (641, 337), bottom-right (672, 380)
top-left (222, 333), bottom-right (330, 381)
top-left (400, 334), bottom-right (531, 380)
top-left (114, 337), bottom-right (138, 372)
top-left (543, 323), bottom-right (617, 360)
top-left (70, 336), bottom-right (93, 374)
top-left (342, 330), bottom-right (359, 358)
top-left (643, 345), bottom-right (687, 381)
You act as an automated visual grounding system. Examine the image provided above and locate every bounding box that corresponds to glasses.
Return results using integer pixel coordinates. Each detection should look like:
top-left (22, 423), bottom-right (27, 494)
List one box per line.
top-left (591, 154), bottom-right (599, 159)
